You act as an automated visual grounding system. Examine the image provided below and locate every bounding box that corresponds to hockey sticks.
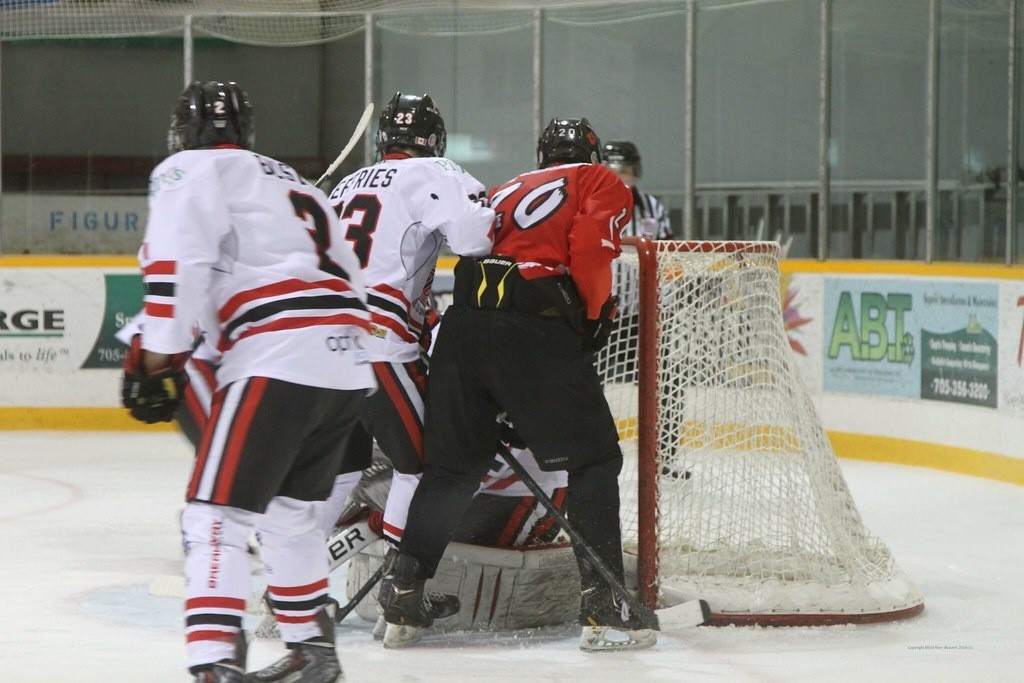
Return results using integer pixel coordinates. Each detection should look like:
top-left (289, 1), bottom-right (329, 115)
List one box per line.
top-left (174, 404), bottom-right (353, 683)
top-left (497, 440), bottom-right (712, 632)
top-left (325, 566), bottom-right (382, 624)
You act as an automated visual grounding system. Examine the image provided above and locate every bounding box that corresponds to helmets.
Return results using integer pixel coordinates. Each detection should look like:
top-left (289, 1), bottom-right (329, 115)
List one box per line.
top-left (537, 118), bottom-right (598, 169)
top-left (377, 91), bottom-right (447, 157)
top-left (166, 80), bottom-right (254, 153)
top-left (601, 139), bottom-right (639, 164)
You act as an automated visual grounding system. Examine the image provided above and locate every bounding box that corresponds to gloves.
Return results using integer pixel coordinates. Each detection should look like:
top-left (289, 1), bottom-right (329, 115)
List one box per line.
top-left (420, 308), bottom-right (442, 351)
top-left (121, 332), bottom-right (185, 423)
top-left (590, 296), bottom-right (620, 353)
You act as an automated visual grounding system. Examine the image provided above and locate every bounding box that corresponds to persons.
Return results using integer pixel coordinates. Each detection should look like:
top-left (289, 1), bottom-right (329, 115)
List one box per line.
top-left (120, 80), bottom-right (379, 683)
top-left (330, 93), bottom-right (708, 653)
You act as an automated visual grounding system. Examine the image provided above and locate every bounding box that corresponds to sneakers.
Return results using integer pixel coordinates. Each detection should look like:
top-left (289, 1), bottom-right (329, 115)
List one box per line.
top-left (577, 556), bottom-right (657, 651)
top-left (247, 598), bottom-right (344, 682)
top-left (373, 549), bottom-right (461, 635)
top-left (384, 554), bottom-right (435, 647)
top-left (194, 628), bottom-right (249, 682)
